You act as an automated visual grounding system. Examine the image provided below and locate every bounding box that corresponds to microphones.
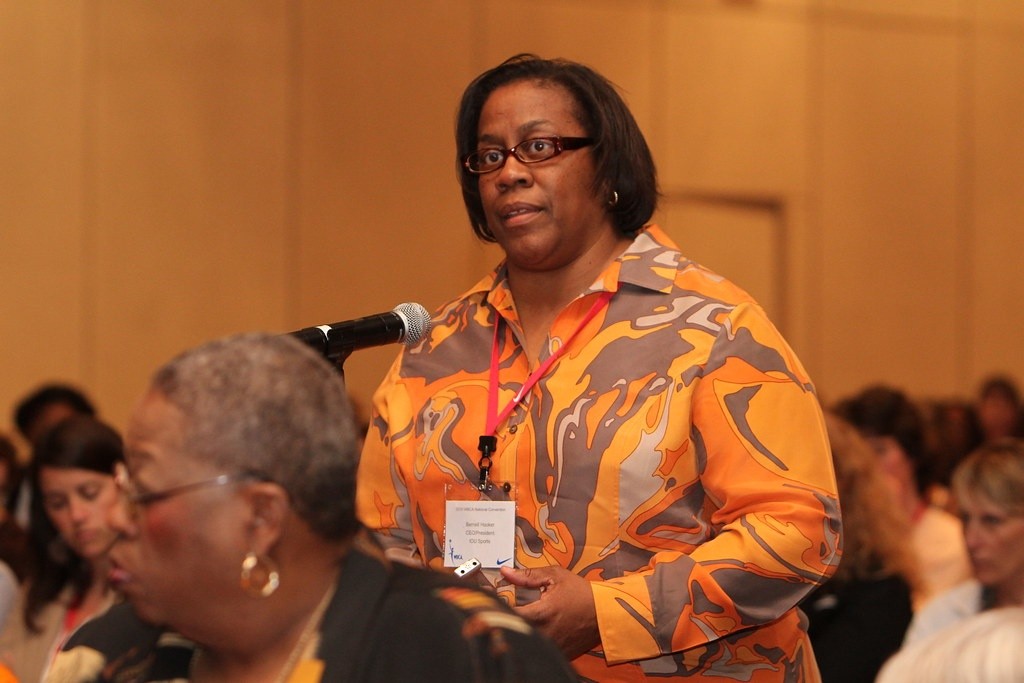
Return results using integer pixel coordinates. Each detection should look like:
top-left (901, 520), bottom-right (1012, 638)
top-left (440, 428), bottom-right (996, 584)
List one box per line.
top-left (286, 302), bottom-right (433, 357)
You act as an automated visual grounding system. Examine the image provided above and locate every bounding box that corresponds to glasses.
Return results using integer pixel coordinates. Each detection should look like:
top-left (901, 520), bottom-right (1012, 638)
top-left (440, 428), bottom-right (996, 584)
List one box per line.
top-left (465, 135), bottom-right (593, 173)
top-left (113, 462), bottom-right (293, 520)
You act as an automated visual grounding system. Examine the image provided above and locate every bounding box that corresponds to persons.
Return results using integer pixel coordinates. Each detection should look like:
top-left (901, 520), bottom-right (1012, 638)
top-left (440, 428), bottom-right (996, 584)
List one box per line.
top-left (356, 53), bottom-right (843, 683)
top-left (802, 376), bottom-right (1024, 683)
top-left (42, 332), bottom-right (596, 683)
top-left (0, 383), bottom-right (134, 683)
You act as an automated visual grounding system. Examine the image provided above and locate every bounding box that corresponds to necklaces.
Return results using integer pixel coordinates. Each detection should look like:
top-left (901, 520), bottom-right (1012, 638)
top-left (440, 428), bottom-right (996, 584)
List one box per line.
top-left (278, 580), bottom-right (338, 682)
